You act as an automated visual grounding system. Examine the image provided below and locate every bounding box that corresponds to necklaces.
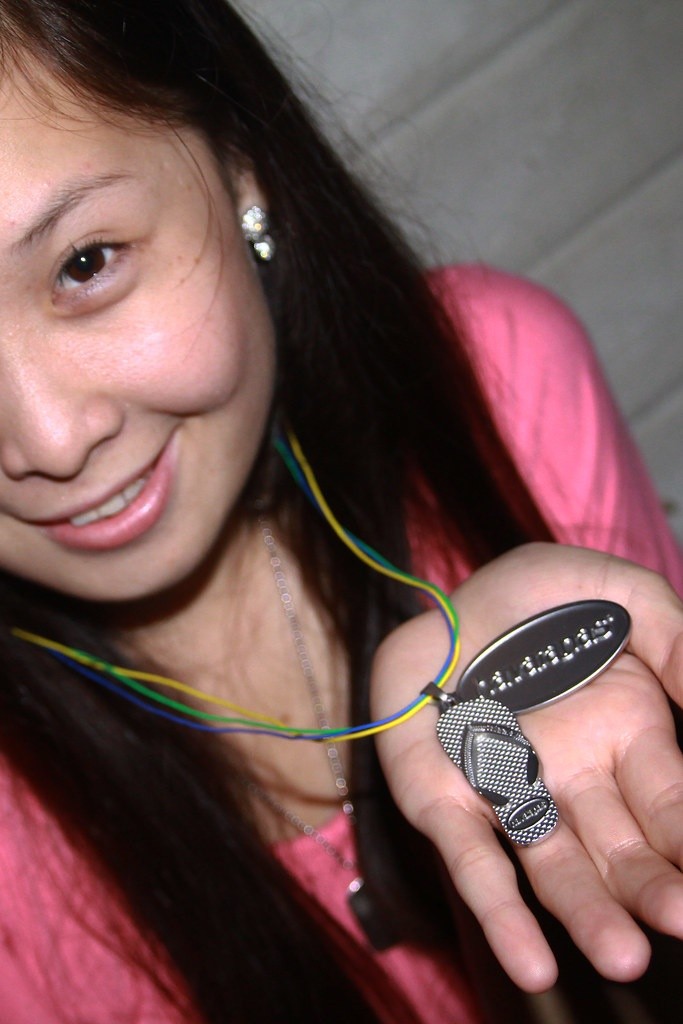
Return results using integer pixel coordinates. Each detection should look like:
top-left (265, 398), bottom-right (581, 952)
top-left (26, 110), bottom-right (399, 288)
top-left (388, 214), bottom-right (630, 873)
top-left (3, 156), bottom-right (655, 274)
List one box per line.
top-left (4, 429), bottom-right (637, 953)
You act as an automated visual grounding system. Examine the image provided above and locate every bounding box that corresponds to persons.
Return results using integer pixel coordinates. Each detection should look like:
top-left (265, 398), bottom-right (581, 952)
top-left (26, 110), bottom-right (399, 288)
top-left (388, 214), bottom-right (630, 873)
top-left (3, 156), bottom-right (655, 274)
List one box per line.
top-left (1, 0), bottom-right (683, 1024)
